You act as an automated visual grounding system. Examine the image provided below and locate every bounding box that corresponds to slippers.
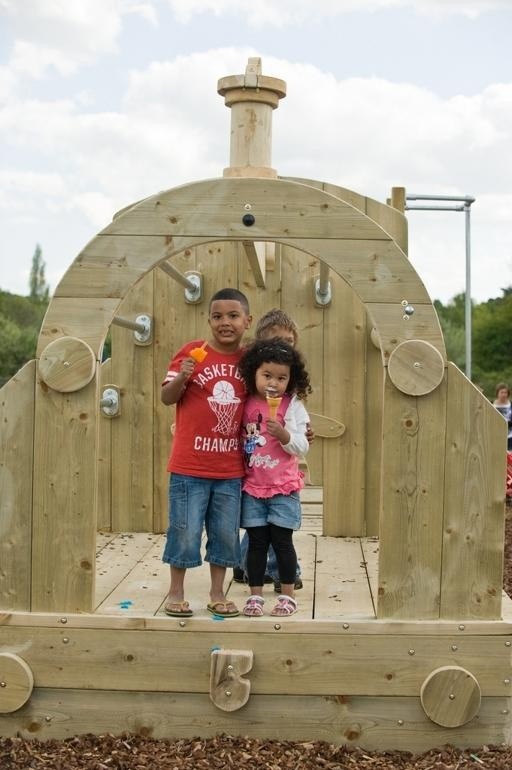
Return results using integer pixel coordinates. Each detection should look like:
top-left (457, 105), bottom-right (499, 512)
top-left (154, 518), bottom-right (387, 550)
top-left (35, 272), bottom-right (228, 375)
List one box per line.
top-left (205, 599), bottom-right (241, 618)
top-left (163, 596), bottom-right (195, 618)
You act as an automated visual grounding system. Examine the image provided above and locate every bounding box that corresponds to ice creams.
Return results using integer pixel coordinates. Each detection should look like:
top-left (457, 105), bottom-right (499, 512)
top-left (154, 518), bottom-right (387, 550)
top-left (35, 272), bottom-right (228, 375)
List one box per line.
top-left (266, 386), bottom-right (282, 420)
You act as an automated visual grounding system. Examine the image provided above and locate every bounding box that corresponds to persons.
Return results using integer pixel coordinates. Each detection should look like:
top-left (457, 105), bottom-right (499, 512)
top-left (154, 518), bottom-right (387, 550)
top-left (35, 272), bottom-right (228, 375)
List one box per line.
top-left (238, 336), bottom-right (312, 617)
top-left (161, 287), bottom-right (316, 617)
top-left (233, 309), bottom-right (303, 592)
top-left (490, 382), bottom-right (512, 450)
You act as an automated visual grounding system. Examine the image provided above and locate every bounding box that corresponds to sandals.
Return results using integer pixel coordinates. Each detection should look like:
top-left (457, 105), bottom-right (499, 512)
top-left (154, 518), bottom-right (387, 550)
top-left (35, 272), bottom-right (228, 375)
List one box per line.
top-left (242, 594), bottom-right (266, 617)
top-left (270, 594), bottom-right (300, 618)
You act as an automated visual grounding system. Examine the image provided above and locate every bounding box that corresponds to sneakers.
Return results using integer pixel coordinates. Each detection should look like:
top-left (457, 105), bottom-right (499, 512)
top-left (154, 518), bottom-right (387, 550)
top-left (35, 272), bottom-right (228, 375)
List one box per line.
top-left (230, 562), bottom-right (274, 585)
top-left (273, 573), bottom-right (305, 594)
